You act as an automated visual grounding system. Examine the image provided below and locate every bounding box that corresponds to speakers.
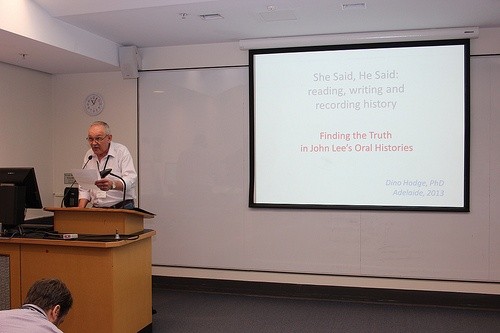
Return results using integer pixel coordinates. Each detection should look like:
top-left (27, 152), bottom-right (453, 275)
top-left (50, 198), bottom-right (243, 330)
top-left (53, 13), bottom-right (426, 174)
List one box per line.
top-left (118, 46), bottom-right (139, 78)
top-left (0, 183), bottom-right (24, 225)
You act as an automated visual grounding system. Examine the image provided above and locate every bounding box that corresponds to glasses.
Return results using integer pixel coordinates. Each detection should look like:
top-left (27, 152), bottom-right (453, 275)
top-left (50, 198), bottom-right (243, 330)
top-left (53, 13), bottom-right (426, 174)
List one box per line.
top-left (85, 135), bottom-right (108, 143)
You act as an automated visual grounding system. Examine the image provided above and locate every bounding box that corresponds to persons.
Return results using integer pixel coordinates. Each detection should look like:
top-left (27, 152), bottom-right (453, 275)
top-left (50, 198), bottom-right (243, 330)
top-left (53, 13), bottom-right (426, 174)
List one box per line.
top-left (78, 121), bottom-right (137, 209)
top-left (0, 278), bottom-right (73, 333)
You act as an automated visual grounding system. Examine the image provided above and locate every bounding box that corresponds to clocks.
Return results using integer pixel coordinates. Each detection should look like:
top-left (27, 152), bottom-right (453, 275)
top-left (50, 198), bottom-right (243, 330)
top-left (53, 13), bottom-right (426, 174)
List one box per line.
top-left (84, 93), bottom-right (104, 116)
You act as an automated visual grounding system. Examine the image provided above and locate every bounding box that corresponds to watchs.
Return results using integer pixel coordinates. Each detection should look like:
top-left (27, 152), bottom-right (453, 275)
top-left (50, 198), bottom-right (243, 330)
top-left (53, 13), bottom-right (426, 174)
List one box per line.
top-left (110, 181), bottom-right (116, 190)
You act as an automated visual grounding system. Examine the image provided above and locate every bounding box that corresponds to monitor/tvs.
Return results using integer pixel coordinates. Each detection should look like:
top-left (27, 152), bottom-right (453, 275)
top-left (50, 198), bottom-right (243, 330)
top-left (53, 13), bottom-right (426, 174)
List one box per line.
top-left (0, 167), bottom-right (43, 209)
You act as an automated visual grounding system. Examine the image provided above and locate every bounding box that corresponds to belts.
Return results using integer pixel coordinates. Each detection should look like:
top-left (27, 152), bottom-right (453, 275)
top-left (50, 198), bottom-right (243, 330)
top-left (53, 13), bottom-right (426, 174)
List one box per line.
top-left (110, 199), bottom-right (133, 209)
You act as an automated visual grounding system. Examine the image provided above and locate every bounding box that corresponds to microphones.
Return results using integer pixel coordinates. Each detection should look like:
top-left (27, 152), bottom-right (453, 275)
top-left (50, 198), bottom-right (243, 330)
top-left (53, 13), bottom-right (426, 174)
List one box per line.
top-left (61, 156), bottom-right (92, 208)
